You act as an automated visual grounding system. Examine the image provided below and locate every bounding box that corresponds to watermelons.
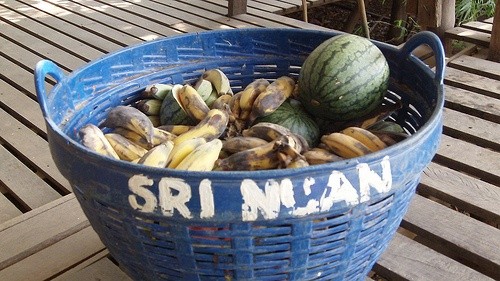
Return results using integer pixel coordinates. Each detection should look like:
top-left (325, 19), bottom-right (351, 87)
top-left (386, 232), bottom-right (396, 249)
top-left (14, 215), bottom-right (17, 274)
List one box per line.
top-left (253, 97), bottom-right (320, 149)
top-left (298, 34), bottom-right (390, 123)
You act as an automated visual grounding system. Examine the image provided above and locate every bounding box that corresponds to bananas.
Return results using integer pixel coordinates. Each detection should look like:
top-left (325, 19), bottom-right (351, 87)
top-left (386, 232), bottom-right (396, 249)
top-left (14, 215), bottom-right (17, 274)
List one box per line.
top-left (218, 76), bottom-right (309, 172)
top-left (304, 104), bottom-right (404, 168)
top-left (81, 69), bottom-right (231, 177)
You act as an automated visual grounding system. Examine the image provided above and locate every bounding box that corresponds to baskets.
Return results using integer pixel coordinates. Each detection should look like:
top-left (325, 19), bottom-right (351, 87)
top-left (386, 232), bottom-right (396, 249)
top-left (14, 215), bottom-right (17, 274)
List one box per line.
top-left (34, 28), bottom-right (445, 280)
